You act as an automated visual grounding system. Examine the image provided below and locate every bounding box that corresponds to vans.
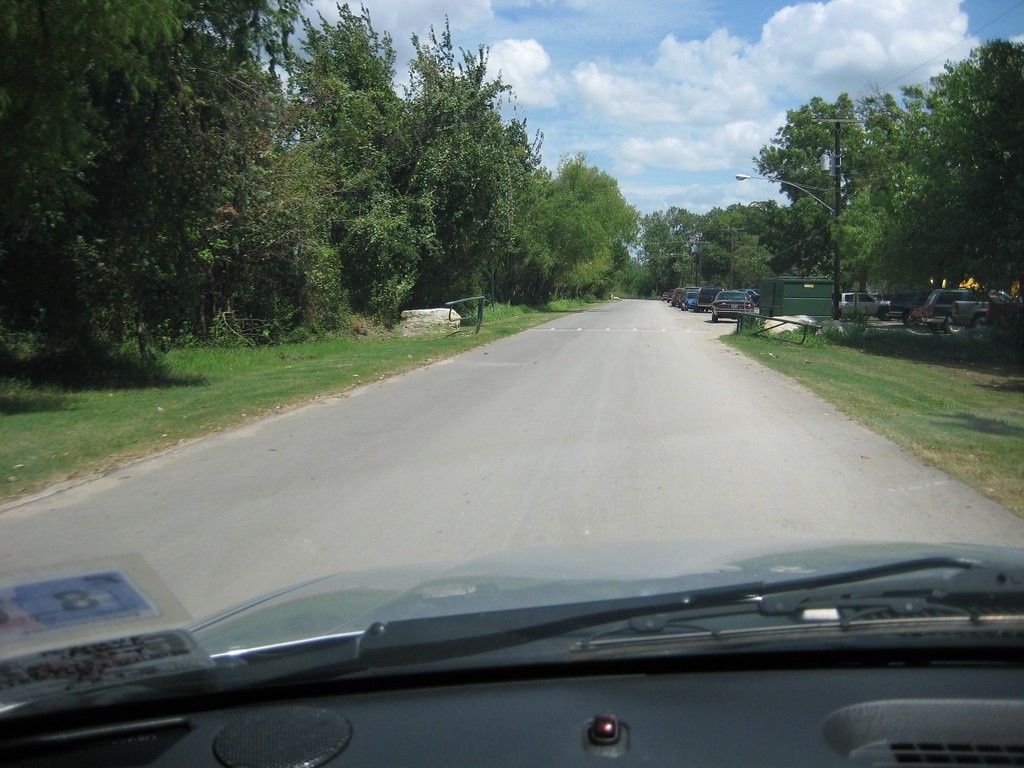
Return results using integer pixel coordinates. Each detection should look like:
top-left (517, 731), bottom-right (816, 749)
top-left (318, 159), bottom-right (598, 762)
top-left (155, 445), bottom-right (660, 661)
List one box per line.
top-left (924, 288), bottom-right (1010, 334)
top-left (883, 289), bottom-right (928, 320)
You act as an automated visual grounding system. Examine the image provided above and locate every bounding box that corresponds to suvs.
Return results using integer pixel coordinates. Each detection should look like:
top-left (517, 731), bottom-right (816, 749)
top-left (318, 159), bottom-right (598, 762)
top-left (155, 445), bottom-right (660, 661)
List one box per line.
top-left (695, 286), bottom-right (724, 311)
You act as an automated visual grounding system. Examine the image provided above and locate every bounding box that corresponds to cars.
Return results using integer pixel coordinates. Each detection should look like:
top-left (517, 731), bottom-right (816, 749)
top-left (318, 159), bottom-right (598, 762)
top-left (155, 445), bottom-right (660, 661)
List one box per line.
top-left (680, 288), bottom-right (698, 311)
top-left (671, 289), bottom-right (683, 306)
top-left (738, 288), bottom-right (761, 303)
top-left (711, 292), bottom-right (755, 323)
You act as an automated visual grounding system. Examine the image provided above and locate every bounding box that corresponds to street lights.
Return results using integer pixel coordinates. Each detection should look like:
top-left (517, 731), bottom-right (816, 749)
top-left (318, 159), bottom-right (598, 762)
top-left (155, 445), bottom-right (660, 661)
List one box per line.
top-left (735, 173), bottom-right (842, 320)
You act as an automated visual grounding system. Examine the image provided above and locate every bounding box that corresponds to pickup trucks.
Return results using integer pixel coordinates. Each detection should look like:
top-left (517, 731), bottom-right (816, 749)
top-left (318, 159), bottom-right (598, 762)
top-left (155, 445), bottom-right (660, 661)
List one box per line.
top-left (837, 292), bottom-right (889, 319)
top-left (951, 292), bottom-right (1024, 330)
